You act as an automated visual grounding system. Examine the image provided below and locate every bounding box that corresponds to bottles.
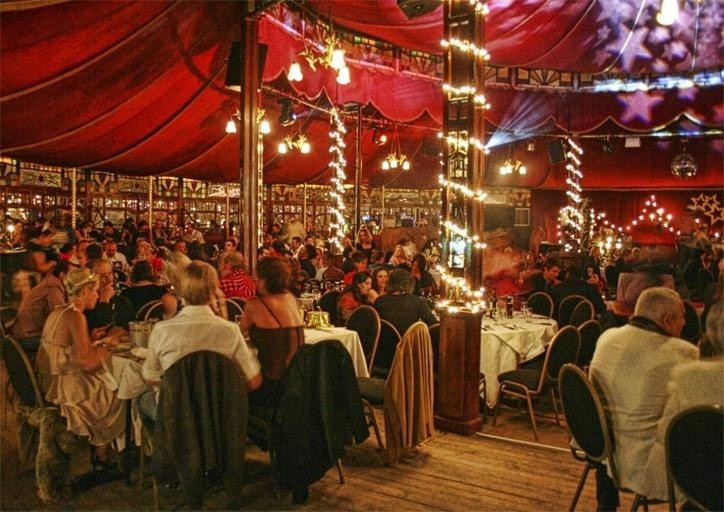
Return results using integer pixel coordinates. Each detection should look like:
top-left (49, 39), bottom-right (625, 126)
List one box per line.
top-left (489, 288), bottom-right (498, 318)
top-left (418, 289), bottom-right (493, 333)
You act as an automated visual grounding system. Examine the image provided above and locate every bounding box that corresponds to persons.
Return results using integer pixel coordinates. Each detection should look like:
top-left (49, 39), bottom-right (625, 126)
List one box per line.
top-left (0, 207), bottom-right (437, 510)
top-left (486, 227), bottom-right (724, 512)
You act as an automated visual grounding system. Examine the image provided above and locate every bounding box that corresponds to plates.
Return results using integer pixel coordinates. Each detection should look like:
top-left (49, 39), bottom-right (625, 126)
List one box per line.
top-left (93, 331), bottom-right (148, 360)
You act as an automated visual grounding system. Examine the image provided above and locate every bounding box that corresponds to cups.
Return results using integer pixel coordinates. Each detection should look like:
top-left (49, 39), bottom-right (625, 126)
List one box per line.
top-left (500, 301), bottom-right (532, 323)
top-left (235, 314), bottom-right (241, 324)
top-left (307, 310), bottom-right (329, 329)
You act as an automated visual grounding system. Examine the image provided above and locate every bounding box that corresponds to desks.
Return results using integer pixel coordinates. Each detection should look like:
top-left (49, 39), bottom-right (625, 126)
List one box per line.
top-left (436, 306), bottom-right (558, 416)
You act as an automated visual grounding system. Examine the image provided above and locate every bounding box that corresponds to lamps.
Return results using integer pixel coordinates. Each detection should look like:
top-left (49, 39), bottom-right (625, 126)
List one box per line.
top-left (437, 131), bottom-right (490, 156)
top-left (470, 1), bottom-right (492, 16)
top-left (278, 134), bottom-right (313, 157)
top-left (441, 82), bottom-right (491, 111)
top-left (274, 97), bottom-right (298, 127)
top-left (441, 38), bottom-right (490, 62)
top-left (286, 36), bottom-right (351, 86)
top-left (370, 122), bottom-right (389, 147)
top-left (379, 151), bottom-right (410, 172)
top-left (224, 107), bottom-right (271, 136)
top-left (499, 156), bottom-right (527, 175)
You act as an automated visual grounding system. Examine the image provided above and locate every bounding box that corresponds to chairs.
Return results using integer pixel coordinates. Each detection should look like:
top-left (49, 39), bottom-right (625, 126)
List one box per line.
top-left (524, 291), bottom-right (603, 360)
top-left (127, 296), bottom-right (250, 331)
top-left (353, 321), bottom-right (432, 466)
top-left (664, 404), bottom-right (723, 510)
top-left (137, 348), bottom-right (249, 510)
top-left (557, 362), bottom-right (650, 512)
top-left (1, 334), bottom-right (124, 502)
top-left (344, 304), bottom-right (381, 375)
top-left (493, 324), bottom-right (582, 441)
top-left (246, 339), bottom-right (346, 502)
